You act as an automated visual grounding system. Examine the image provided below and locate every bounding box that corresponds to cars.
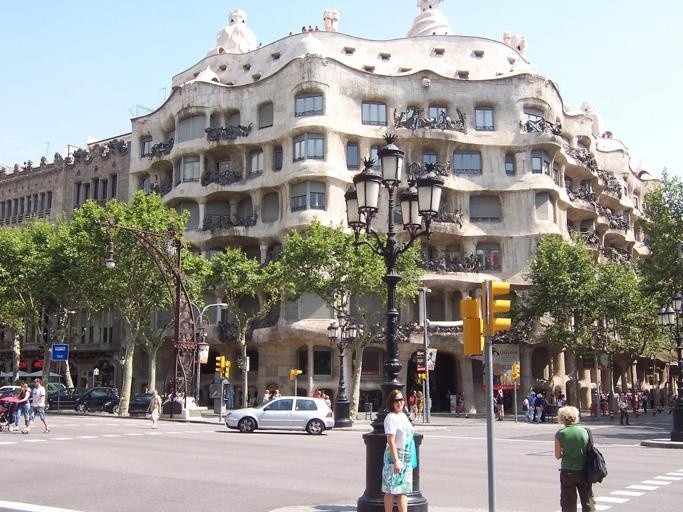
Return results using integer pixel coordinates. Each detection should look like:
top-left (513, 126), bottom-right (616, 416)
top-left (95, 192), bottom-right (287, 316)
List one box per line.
top-left (45, 388), bottom-right (91, 412)
top-left (621, 392), bottom-right (641, 411)
top-left (0, 382), bottom-right (67, 414)
top-left (223, 396), bottom-right (336, 434)
top-left (75, 386), bottom-right (120, 415)
top-left (102, 392), bottom-right (155, 415)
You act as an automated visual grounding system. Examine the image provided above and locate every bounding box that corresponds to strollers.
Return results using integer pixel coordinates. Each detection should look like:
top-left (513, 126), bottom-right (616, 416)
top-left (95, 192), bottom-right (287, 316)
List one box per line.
top-left (0, 396), bottom-right (21, 432)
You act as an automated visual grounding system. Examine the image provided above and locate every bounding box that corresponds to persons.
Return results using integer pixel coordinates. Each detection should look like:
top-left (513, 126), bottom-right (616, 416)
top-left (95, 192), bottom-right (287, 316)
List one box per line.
top-left (323, 395), bottom-right (331, 410)
top-left (145, 390), bottom-right (161, 429)
top-left (554, 406), bottom-right (598, 511)
top-left (272, 389), bottom-right (280, 399)
top-left (307, 25), bottom-right (314, 33)
top-left (455, 390), bottom-right (469, 418)
top-left (319, 392), bottom-right (326, 400)
top-left (262, 389), bottom-right (271, 403)
top-left (302, 27), bottom-right (306, 33)
top-left (313, 391), bottom-right (320, 399)
top-left (10, 383), bottom-right (30, 431)
top-left (491, 347), bottom-right (503, 386)
top-left (314, 26), bottom-right (319, 33)
top-left (381, 389), bottom-right (414, 511)
top-left (408, 390), bottom-right (417, 421)
top-left (494, 389), bottom-right (505, 421)
top-left (21, 377), bottom-right (50, 434)
top-left (521, 386), bottom-right (679, 426)
top-left (416, 391), bottom-right (424, 422)
top-left (445, 389), bottom-right (450, 413)
top-left (0, 403), bottom-right (6, 416)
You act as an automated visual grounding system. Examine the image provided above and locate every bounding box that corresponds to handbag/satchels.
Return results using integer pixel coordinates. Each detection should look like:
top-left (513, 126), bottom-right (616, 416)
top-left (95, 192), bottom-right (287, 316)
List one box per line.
top-left (586, 447), bottom-right (607, 483)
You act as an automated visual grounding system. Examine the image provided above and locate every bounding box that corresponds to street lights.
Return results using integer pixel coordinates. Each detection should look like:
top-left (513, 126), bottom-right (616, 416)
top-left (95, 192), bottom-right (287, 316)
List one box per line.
top-left (179, 299), bottom-right (228, 407)
top-left (409, 286), bottom-right (435, 424)
top-left (295, 369), bottom-right (303, 377)
top-left (104, 219), bottom-right (197, 414)
top-left (325, 309), bottom-right (360, 426)
top-left (343, 129), bottom-right (449, 512)
top-left (657, 292), bottom-right (683, 442)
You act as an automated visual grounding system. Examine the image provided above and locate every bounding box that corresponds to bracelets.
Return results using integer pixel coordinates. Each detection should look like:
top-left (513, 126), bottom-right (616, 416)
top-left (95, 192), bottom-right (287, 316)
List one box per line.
top-left (393, 458), bottom-right (398, 461)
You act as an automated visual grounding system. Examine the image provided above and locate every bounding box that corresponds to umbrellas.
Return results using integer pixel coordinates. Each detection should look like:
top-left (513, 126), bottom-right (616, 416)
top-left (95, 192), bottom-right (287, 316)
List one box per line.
top-left (23, 369), bottom-right (63, 377)
top-left (0, 370), bottom-right (29, 379)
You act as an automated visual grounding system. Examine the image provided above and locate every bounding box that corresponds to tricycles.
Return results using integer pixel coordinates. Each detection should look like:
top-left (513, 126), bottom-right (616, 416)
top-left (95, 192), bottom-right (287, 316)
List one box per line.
top-left (590, 395), bottom-right (609, 417)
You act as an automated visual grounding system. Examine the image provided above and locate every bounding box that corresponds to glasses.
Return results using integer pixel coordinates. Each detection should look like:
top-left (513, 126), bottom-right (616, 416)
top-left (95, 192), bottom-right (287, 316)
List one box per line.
top-left (393, 397), bottom-right (405, 402)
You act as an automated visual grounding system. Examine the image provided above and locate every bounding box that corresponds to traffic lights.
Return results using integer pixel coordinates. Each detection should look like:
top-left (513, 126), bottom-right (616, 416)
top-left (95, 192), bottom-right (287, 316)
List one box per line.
top-left (513, 363), bottom-right (521, 381)
top-left (484, 278), bottom-right (510, 337)
top-left (216, 356), bottom-right (225, 373)
